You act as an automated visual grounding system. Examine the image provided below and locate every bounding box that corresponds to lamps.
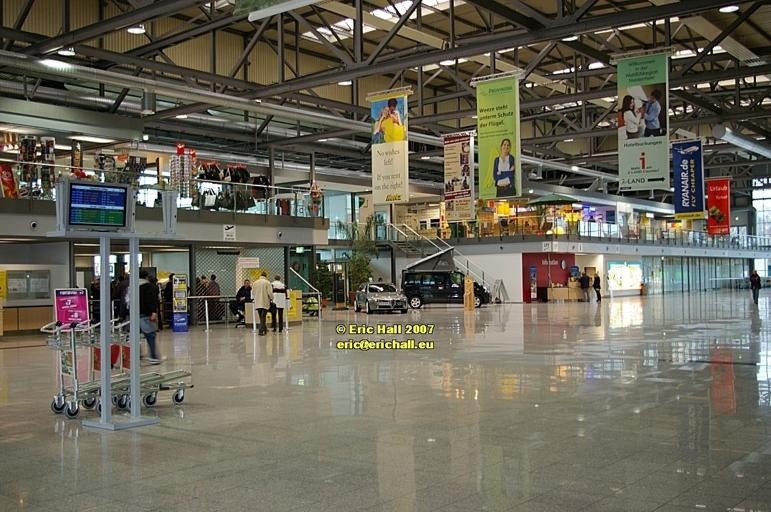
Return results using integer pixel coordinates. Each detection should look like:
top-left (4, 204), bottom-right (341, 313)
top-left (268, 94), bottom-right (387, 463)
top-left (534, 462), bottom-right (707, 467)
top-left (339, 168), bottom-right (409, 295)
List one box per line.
top-left (127, 23), bottom-right (146, 34)
top-left (338, 79), bottom-right (352, 86)
top-left (57, 44), bottom-right (75, 56)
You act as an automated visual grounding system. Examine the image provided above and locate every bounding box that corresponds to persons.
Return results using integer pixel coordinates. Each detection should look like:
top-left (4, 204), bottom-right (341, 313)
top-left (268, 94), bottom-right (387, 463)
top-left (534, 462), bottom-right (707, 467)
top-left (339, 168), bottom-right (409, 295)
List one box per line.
top-left (374, 106), bottom-right (405, 144)
top-left (581, 304), bottom-right (590, 327)
top-left (270, 275), bottom-right (289, 334)
top-left (388, 97), bottom-right (402, 126)
top-left (622, 95), bottom-right (646, 139)
top-left (639, 90), bottom-right (665, 138)
top-left (593, 273), bottom-right (602, 302)
top-left (579, 271), bottom-right (591, 302)
top-left (271, 333), bottom-right (290, 381)
top-left (196, 277), bottom-right (207, 325)
top-left (252, 333), bottom-right (271, 379)
top-left (229, 279), bottom-right (254, 323)
top-left (750, 269), bottom-right (764, 304)
top-left (302, 294), bottom-right (321, 316)
top-left (199, 275), bottom-right (210, 289)
top-left (208, 331), bottom-right (223, 366)
top-left (593, 302), bottom-right (602, 328)
top-left (752, 303), bottom-right (764, 335)
top-left (124, 270), bottom-right (169, 367)
top-left (230, 335), bottom-right (252, 371)
top-left (206, 273), bottom-right (220, 321)
top-left (90, 272), bottom-right (190, 333)
top-left (493, 137), bottom-right (518, 198)
top-left (251, 271), bottom-right (275, 337)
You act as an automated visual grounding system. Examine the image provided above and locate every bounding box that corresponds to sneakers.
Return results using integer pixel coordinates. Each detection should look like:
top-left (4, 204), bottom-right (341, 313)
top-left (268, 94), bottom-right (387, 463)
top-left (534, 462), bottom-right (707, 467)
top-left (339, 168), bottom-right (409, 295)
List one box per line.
top-left (155, 356), bottom-right (165, 364)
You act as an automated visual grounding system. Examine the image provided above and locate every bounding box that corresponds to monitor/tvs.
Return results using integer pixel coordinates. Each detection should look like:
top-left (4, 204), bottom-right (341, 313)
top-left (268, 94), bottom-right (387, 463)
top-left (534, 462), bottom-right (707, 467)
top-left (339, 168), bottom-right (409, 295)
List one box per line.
top-left (56, 178), bottom-right (131, 231)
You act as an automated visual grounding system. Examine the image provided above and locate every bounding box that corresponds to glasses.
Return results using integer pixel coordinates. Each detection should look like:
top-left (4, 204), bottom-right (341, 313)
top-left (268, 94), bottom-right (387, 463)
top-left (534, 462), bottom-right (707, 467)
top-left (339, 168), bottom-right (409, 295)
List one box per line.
top-left (168, 155), bottom-right (198, 199)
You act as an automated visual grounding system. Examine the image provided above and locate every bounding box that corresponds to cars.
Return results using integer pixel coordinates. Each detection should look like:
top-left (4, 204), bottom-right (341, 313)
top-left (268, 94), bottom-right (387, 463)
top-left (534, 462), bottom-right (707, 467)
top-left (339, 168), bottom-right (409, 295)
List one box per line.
top-left (351, 282), bottom-right (409, 316)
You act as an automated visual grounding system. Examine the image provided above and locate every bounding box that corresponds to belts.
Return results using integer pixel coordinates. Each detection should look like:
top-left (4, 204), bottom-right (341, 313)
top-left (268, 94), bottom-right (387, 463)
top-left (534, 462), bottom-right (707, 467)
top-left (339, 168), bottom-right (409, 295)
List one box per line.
top-left (496, 184), bottom-right (511, 191)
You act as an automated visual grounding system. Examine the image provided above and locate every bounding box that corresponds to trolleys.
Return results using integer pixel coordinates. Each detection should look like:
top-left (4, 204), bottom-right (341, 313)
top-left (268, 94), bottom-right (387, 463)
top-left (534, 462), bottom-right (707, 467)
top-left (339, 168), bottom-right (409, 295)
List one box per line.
top-left (39, 318), bottom-right (195, 420)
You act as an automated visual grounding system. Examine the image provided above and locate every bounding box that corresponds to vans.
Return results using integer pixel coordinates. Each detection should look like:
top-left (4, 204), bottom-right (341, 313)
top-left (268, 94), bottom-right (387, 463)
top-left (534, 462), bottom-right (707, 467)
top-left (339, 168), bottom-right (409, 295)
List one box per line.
top-left (400, 270), bottom-right (493, 309)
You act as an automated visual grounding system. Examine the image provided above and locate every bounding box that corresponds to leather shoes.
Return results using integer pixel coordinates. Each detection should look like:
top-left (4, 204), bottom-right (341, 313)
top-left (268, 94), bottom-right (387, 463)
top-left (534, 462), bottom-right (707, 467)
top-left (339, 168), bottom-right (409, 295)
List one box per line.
top-left (259, 328), bottom-right (282, 336)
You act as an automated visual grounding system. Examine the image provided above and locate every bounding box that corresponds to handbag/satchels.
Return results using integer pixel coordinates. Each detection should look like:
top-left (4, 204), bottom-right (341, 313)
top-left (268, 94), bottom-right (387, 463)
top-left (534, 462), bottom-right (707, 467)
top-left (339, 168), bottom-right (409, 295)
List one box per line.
top-left (191, 161), bottom-right (255, 211)
top-left (268, 300), bottom-right (277, 313)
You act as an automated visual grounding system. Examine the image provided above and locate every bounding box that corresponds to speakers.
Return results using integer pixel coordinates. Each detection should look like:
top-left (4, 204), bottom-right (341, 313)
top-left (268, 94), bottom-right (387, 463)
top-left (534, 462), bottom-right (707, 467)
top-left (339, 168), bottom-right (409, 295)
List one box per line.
top-left (138, 266), bottom-right (157, 283)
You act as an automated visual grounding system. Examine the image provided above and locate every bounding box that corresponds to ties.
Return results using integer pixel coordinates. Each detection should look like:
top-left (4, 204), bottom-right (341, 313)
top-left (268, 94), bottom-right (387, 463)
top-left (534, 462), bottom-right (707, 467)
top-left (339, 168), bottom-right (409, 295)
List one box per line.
top-left (645, 103), bottom-right (652, 126)
